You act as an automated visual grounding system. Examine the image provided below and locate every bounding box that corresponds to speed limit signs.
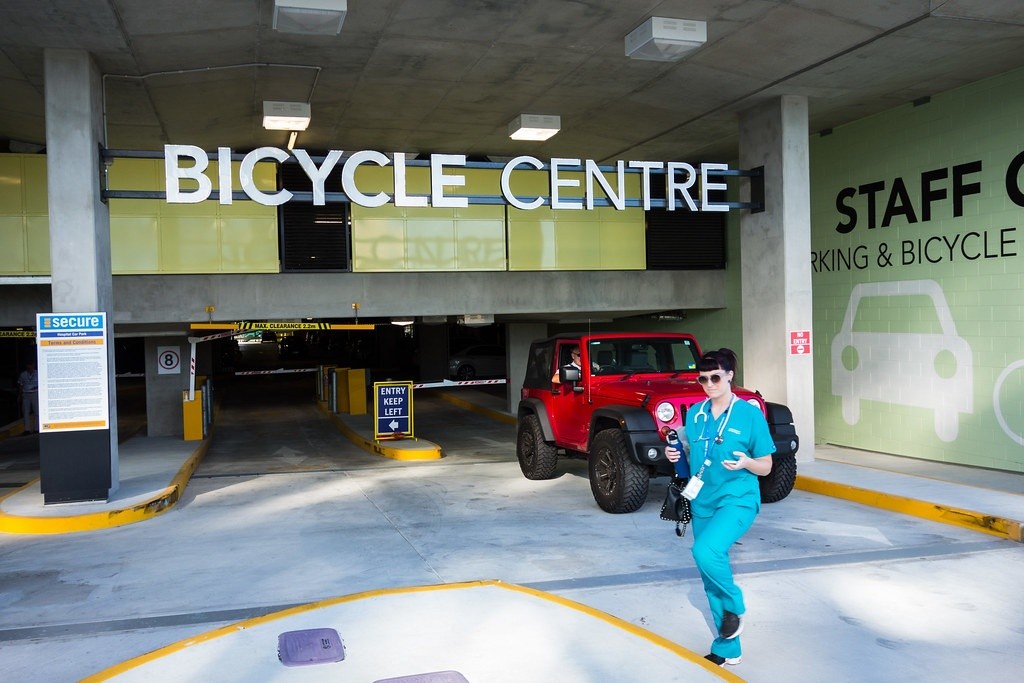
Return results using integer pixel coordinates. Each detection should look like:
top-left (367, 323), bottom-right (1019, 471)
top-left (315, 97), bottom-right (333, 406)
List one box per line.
top-left (158, 351), bottom-right (181, 370)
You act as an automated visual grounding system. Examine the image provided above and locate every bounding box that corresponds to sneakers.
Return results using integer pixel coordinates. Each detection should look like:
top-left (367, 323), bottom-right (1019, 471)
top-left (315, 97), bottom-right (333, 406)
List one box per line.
top-left (718, 611), bottom-right (745, 640)
top-left (704, 653), bottom-right (742, 668)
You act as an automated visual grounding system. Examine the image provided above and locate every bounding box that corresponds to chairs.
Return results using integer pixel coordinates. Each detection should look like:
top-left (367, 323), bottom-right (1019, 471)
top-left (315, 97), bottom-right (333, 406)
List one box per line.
top-left (596, 350), bottom-right (613, 367)
top-left (623, 352), bottom-right (656, 373)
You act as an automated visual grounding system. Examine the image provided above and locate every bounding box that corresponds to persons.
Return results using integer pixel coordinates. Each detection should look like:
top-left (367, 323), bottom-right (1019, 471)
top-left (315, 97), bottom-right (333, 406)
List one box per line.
top-left (665, 348), bottom-right (777, 668)
top-left (551, 346), bottom-right (600, 384)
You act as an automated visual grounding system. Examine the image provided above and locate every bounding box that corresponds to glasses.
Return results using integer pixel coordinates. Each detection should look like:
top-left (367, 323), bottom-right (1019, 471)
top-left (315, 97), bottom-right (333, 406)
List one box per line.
top-left (697, 372), bottom-right (726, 386)
top-left (572, 352), bottom-right (580, 357)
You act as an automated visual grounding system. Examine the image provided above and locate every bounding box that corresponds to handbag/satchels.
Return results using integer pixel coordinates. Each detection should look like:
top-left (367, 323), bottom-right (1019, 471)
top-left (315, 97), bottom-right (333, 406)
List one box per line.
top-left (659, 477), bottom-right (692, 538)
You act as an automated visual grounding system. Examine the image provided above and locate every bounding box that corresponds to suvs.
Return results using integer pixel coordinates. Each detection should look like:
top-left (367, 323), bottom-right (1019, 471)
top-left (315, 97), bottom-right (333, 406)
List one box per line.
top-left (515, 331), bottom-right (800, 514)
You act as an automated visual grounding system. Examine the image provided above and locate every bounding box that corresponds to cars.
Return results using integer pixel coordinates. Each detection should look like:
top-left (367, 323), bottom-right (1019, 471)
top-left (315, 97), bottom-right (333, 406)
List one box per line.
top-left (448, 344), bottom-right (506, 381)
